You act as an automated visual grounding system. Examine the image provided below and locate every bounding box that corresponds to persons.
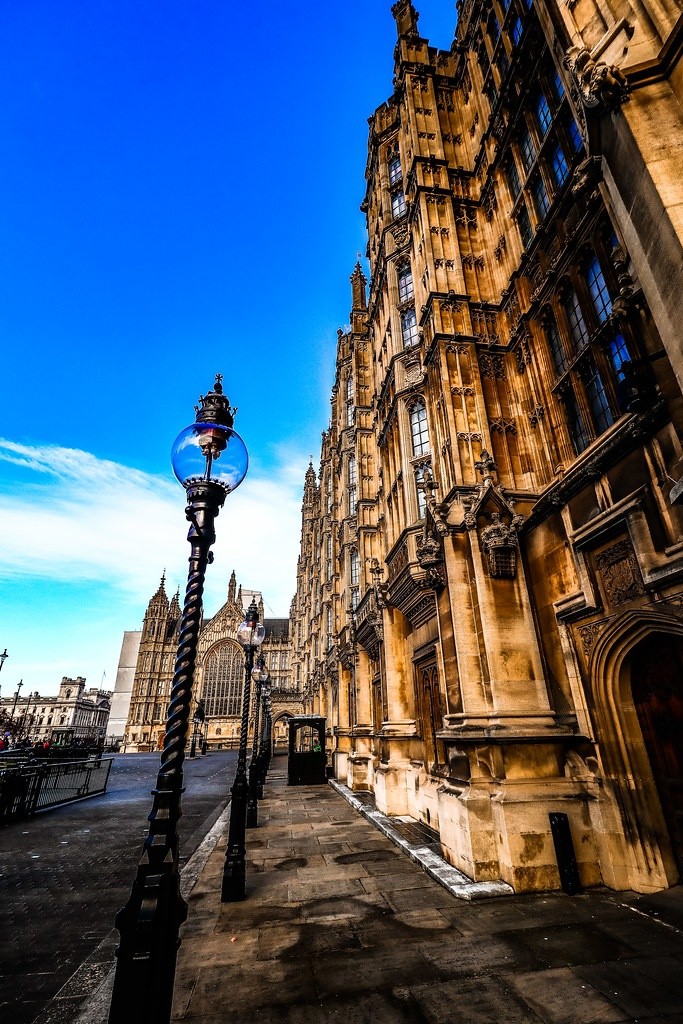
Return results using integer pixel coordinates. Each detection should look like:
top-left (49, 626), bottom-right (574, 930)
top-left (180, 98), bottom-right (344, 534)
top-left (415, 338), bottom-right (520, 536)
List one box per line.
top-left (21, 737), bottom-right (43, 748)
top-left (43, 737), bottom-right (63, 750)
top-left (273, 736), bottom-right (276, 754)
top-left (314, 740), bottom-right (321, 752)
top-left (0, 738), bottom-right (10, 751)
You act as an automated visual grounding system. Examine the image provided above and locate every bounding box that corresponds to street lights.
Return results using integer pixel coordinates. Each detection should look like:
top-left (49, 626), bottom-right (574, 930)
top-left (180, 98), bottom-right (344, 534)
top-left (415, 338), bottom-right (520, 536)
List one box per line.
top-left (10, 678), bottom-right (33, 737)
top-left (190, 698), bottom-right (204, 758)
top-left (108, 375), bottom-right (250, 1024)
top-left (219, 594), bottom-right (275, 902)
top-left (0, 648), bottom-right (9, 672)
